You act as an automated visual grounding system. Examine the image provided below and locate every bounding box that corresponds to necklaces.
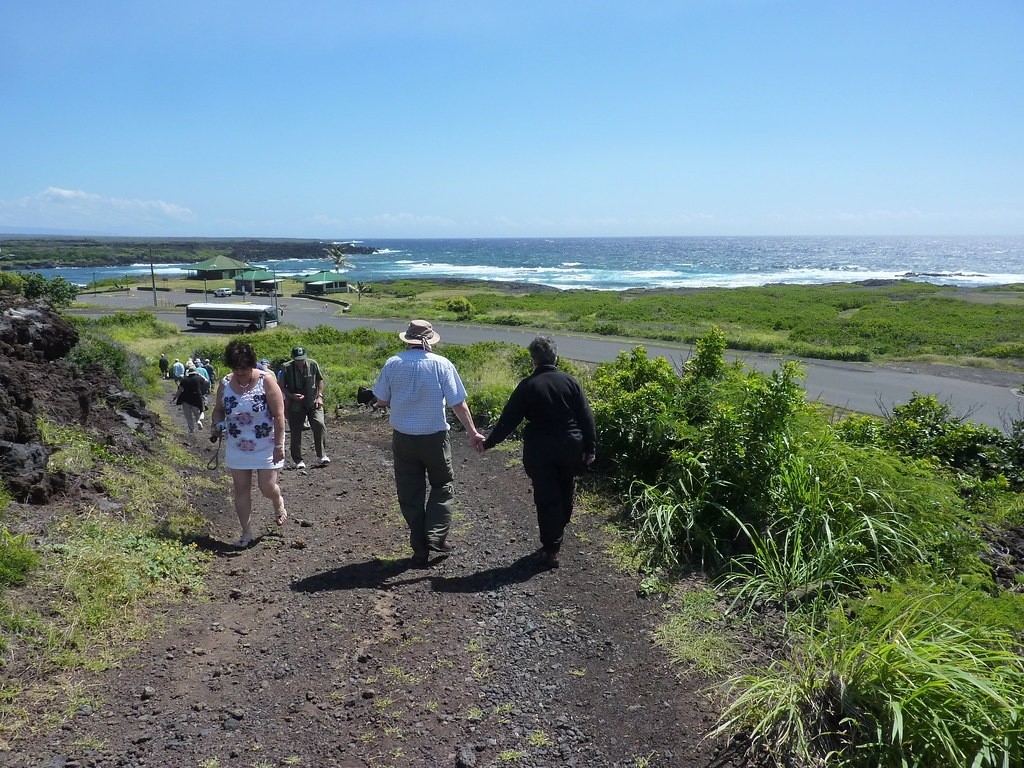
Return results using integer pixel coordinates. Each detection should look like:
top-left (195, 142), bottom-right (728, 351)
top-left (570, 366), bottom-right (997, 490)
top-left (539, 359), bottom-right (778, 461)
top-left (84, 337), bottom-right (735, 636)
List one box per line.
top-left (237, 368), bottom-right (253, 388)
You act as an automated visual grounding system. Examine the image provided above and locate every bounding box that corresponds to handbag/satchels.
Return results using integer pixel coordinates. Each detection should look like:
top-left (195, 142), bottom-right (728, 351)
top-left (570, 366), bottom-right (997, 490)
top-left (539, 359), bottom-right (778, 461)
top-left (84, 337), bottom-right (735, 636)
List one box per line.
top-left (557, 382), bottom-right (589, 474)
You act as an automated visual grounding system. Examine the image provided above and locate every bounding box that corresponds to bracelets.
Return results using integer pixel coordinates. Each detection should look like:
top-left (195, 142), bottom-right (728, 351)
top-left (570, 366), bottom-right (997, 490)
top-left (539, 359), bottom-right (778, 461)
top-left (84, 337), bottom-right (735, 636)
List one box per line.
top-left (274, 444), bottom-right (284, 449)
top-left (316, 393), bottom-right (322, 398)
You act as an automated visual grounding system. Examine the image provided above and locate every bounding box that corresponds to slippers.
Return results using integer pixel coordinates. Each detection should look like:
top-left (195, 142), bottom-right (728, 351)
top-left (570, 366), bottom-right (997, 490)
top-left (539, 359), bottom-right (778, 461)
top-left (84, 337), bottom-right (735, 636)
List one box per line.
top-left (236, 536), bottom-right (253, 549)
top-left (274, 509), bottom-right (288, 526)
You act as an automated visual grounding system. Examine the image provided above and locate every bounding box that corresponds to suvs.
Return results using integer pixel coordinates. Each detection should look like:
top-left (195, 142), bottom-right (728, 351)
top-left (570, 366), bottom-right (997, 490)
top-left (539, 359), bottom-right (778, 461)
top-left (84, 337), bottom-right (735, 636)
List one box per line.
top-left (213, 288), bottom-right (233, 297)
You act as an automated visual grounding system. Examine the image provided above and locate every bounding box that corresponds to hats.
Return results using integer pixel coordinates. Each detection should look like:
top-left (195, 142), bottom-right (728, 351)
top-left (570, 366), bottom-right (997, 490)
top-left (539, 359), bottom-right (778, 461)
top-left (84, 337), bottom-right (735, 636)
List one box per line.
top-left (261, 359), bottom-right (270, 365)
top-left (196, 359), bottom-right (200, 362)
top-left (175, 359), bottom-right (179, 361)
top-left (161, 354), bottom-right (164, 356)
top-left (291, 346), bottom-right (307, 360)
top-left (205, 359), bottom-right (209, 361)
top-left (399, 320), bottom-right (440, 350)
top-left (187, 368), bottom-right (197, 374)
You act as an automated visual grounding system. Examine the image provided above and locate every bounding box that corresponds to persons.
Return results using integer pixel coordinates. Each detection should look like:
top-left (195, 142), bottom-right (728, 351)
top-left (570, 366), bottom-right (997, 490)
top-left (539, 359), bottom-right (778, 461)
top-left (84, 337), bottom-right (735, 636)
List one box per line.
top-left (373, 319), bottom-right (486, 567)
top-left (475, 336), bottom-right (596, 568)
top-left (210, 340), bottom-right (287, 547)
top-left (159, 346), bottom-right (311, 431)
top-left (280, 346), bottom-right (330, 468)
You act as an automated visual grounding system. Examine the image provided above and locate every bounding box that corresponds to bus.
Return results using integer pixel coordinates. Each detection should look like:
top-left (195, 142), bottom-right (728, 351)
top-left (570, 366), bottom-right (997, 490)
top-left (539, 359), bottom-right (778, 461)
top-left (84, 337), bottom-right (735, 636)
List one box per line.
top-left (186, 302), bottom-right (283, 333)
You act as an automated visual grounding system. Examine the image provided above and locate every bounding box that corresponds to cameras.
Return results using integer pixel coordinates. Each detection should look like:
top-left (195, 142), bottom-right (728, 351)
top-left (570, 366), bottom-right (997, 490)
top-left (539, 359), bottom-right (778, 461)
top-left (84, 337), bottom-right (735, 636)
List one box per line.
top-left (216, 420), bottom-right (229, 431)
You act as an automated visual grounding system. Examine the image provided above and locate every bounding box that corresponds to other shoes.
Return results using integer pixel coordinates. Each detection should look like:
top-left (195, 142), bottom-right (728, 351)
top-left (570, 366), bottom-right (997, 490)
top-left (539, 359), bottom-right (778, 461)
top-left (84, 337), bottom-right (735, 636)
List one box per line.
top-left (543, 536), bottom-right (563, 552)
top-left (317, 455), bottom-right (330, 464)
top-left (412, 555), bottom-right (428, 566)
top-left (198, 420), bottom-right (203, 430)
top-left (205, 404), bottom-right (208, 410)
top-left (295, 460), bottom-right (305, 469)
top-left (190, 429), bottom-right (195, 433)
top-left (427, 537), bottom-right (452, 552)
